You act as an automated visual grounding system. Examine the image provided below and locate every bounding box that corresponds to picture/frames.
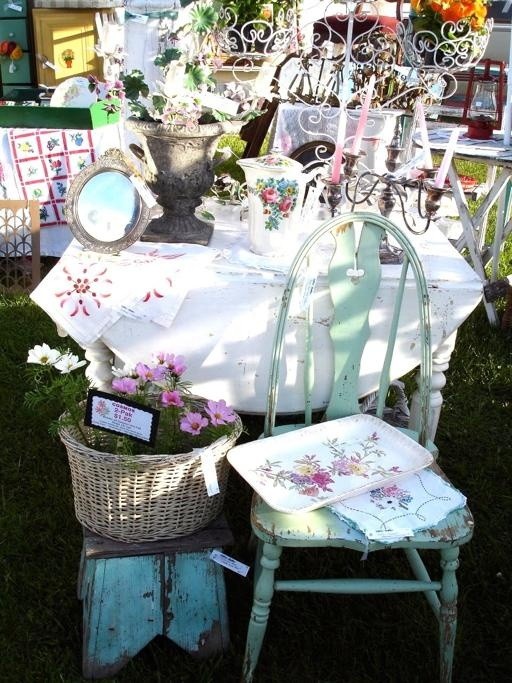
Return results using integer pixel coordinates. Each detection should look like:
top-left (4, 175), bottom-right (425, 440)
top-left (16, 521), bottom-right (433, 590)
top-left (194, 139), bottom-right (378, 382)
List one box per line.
top-left (33, 8), bottom-right (105, 92)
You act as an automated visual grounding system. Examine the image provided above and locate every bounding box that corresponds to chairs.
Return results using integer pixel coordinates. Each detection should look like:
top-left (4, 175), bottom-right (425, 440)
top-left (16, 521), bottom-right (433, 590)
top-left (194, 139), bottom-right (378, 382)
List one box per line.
top-left (312, 0), bottom-right (405, 67)
top-left (242, 212), bottom-right (472, 682)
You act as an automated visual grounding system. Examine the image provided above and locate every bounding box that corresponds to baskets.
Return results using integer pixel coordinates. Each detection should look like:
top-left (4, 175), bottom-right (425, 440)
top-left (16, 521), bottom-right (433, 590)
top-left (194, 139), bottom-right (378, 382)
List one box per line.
top-left (55, 384), bottom-right (244, 545)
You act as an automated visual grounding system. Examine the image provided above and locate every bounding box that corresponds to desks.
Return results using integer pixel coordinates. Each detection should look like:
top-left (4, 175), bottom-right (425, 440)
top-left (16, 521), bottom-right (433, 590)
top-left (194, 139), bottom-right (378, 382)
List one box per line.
top-left (415, 127), bottom-right (512, 327)
top-left (270, 104), bottom-right (415, 177)
top-left (0, 124), bottom-right (126, 260)
top-left (32, 194), bottom-right (484, 444)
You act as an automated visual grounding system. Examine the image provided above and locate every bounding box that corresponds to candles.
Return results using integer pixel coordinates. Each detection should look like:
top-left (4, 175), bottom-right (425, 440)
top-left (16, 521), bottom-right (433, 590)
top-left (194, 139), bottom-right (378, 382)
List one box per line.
top-left (330, 109), bottom-right (348, 184)
top-left (434, 123), bottom-right (460, 190)
top-left (416, 101), bottom-right (433, 170)
top-left (351, 73), bottom-right (377, 156)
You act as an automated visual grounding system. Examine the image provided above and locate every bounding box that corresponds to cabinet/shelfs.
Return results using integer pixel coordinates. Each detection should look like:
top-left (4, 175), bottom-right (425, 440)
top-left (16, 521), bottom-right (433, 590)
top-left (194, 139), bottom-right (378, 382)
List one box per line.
top-left (0, 1), bottom-right (40, 102)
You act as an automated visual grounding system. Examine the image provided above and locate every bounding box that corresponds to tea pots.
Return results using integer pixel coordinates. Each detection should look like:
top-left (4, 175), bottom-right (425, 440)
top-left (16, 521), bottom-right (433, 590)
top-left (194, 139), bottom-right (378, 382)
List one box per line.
top-left (235, 146), bottom-right (326, 259)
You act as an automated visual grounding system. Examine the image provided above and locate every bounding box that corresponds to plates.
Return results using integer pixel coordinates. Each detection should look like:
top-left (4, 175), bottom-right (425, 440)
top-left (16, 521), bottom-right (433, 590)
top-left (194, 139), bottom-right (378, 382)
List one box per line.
top-left (49, 75), bottom-right (98, 110)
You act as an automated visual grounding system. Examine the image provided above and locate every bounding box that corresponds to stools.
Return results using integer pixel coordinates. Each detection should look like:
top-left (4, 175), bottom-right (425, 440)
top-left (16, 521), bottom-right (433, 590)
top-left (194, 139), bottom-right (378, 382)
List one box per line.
top-left (78, 520), bottom-right (237, 680)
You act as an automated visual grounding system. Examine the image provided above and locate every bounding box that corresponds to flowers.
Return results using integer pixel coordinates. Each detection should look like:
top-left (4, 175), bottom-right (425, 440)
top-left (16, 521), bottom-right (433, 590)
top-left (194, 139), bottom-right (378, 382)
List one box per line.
top-left (409, 1), bottom-right (491, 57)
top-left (196, 0), bottom-right (299, 29)
top-left (86, 4), bottom-right (261, 128)
top-left (25, 346), bottom-right (246, 454)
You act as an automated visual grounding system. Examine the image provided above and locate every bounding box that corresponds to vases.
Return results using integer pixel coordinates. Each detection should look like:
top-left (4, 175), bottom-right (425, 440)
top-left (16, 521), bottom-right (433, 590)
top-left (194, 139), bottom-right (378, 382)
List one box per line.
top-left (227, 19), bottom-right (287, 52)
top-left (420, 23), bottom-right (470, 66)
top-left (126, 113), bottom-right (227, 244)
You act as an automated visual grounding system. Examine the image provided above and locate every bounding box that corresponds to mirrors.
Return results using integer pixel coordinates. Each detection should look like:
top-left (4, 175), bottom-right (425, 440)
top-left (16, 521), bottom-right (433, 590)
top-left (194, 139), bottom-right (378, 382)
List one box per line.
top-left (63, 147), bottom-right (152, 254)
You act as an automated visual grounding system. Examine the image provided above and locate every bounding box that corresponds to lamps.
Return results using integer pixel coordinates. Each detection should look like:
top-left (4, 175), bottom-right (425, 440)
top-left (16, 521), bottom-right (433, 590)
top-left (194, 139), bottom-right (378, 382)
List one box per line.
top-left (461, 58), bottom-right (505, 139)
